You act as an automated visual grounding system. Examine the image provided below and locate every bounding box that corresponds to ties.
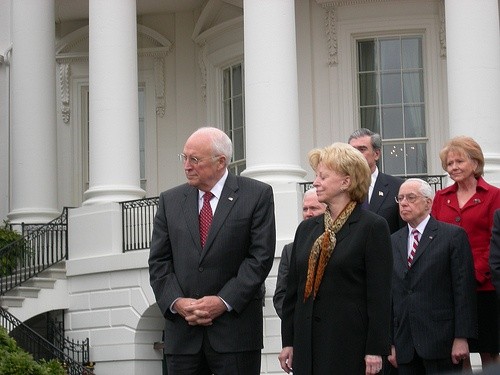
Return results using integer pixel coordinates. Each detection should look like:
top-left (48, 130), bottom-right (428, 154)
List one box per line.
top-left (197, 193), bottom-right (216, 249)
top-left (407, 229), bottom-right (421, 266)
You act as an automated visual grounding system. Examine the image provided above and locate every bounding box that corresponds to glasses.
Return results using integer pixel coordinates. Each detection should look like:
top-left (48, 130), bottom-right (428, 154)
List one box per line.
top-left (178, 152), bottom-right (218, 164)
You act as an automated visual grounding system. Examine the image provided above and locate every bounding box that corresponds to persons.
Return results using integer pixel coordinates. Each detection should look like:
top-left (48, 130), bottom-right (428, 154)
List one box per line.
top-left (347, 128), bottom-right (407, 235)
top-left (272, 187), bottom-right (328, 320)
top-left (387, 177), bottom-right (479, 375)
top-left (277, 141), bottom-right (395, 375)
top-left (488, 208), bottom-right (500, 300)
top-left (429, 136), bottom-right (500, 375)
top-left (147, 126), bottom-right (276, 375)
